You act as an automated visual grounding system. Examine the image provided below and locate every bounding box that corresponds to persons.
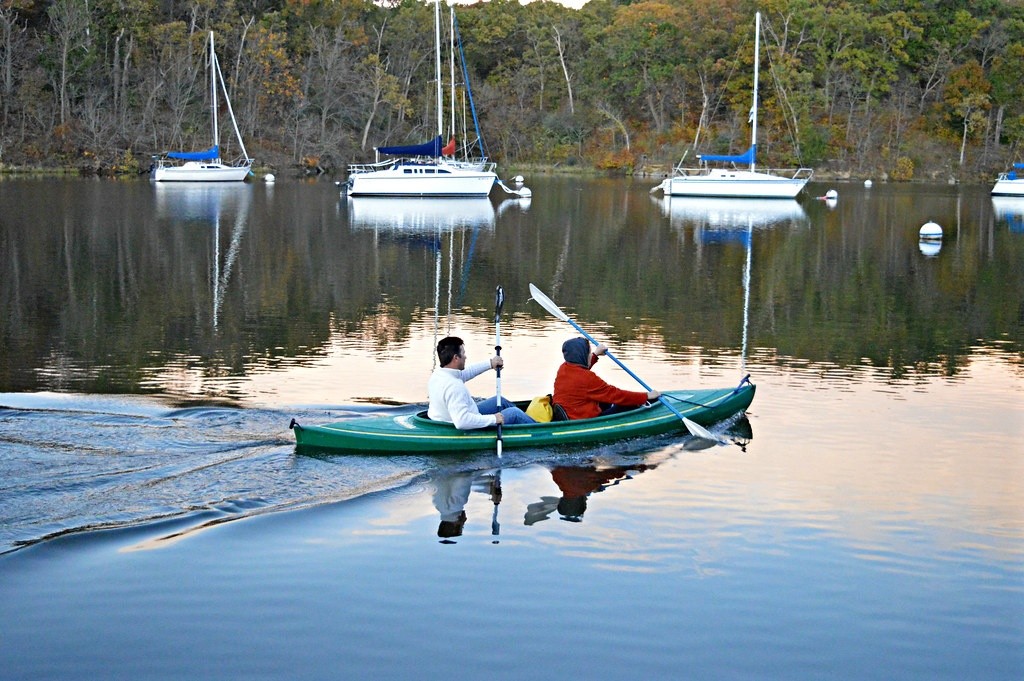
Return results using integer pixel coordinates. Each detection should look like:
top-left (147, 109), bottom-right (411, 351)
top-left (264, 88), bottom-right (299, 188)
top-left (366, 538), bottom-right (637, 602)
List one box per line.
top-left (549, 464), bottom-right (645, 523)
top-left (427, 337), bottom-right (536, 429)
top-left (552, 337), bottom-right (662, 420)
top-left (430, 470), bottom-right (502, 543)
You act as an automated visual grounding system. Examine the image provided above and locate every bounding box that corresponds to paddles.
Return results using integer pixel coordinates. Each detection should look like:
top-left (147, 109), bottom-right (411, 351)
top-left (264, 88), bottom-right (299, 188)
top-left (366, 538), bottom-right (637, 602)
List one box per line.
top-left (523, 436), bottom-right (717, 526)
top-left (496, 284), bottom-right (508, 457)
top-left (492, 465), bottom-right (501, 535)
top-left (529, 281), bottom-right (720, 439)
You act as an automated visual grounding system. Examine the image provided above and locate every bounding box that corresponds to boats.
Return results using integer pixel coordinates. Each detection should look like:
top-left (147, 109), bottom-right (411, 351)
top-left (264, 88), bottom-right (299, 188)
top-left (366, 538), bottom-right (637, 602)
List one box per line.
top-left (991, 162), bottom-right (1024, 197)
top-left (288, 373), bottom-right (757, 453)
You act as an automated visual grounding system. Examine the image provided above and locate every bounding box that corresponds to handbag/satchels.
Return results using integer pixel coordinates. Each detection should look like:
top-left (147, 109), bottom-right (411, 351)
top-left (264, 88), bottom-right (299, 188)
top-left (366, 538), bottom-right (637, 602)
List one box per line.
top-left (525, 396), bottom-right (553, 423)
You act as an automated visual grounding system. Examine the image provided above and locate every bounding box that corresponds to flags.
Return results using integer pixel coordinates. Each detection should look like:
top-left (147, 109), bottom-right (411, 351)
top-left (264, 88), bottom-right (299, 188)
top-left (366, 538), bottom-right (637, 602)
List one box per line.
top-left (443, 137), bottom-right (456, 154)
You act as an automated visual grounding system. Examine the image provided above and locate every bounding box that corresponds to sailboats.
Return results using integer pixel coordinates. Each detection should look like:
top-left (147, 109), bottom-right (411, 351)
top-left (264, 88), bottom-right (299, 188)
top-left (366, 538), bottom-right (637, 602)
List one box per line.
top-left (149, 31), bottom-right (254, 182)
top-left (648, 12), bottom-right (814, 197)
top-left (335, 0), bottom-right (498, 198)
